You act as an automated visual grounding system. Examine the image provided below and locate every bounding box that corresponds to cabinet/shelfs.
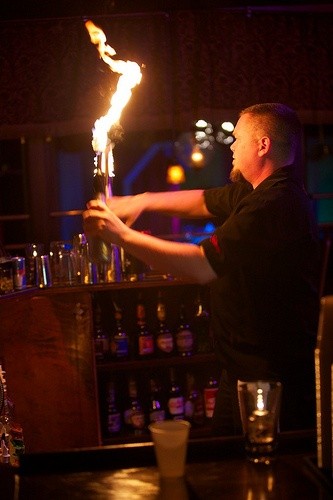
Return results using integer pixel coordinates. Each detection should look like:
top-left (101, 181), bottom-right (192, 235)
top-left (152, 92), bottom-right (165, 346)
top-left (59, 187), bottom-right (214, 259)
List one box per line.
top-left (0, 279), bottom-right (223, 454)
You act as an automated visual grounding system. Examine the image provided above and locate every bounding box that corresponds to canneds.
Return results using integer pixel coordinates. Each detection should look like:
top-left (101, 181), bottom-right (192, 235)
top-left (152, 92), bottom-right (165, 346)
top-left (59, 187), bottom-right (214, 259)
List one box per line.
top-left (34, 254), bottom-right (51, 288)
top-left (10, 257), bottom-right (27, 290)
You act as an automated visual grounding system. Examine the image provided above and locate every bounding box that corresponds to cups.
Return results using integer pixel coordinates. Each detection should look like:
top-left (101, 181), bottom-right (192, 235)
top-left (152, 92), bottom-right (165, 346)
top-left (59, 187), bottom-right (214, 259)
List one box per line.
top-left (149, 420), bottom-right (190, 477)
top-left (28, 232), bottom-right (131, 289)
top-left (237, 377), bottom-right (283, 452)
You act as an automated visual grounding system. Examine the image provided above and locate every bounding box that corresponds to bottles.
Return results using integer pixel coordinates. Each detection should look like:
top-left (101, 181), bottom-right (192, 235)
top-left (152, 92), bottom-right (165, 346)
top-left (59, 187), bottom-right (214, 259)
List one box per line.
top-left (102, 380), bottom-right (122, 439)
top-left (166, 366), bottom-right (185, 420)
top-left (189, 381), bottom-right (204, 416)
top-left (93, 306), bottom-right (109, 364)
top-left (145, 379), bottom-right (164, 422)
top-left (192, 297), bottom-right (212, 355)
top-left (173, 304), bottom-right (194, 359)
top-left (314, 296), bottom-right (333, 470)
top-left (155, 303), bottom-right (175, 361)
top-left (88, 191), bottom-right (114, 265)
top-left (125, 380), bottom-right (146, 443)
top-left (112, 306), bottom-right (131, 362)
top-left (134, 305), bottom-right (154, 362)
top-left (203, 373), bottom-right (220, 420)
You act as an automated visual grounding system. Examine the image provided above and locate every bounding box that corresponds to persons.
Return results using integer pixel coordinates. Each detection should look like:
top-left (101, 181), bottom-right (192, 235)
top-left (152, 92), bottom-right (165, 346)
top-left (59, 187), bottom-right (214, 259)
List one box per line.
top-left (83, 103), bottom-right (323, 430)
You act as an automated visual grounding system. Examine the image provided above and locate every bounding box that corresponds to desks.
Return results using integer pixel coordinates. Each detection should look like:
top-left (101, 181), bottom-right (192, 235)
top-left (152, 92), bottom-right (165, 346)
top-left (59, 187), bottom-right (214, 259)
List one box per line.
top-left (20, 453), bottom-right (333, 500)
